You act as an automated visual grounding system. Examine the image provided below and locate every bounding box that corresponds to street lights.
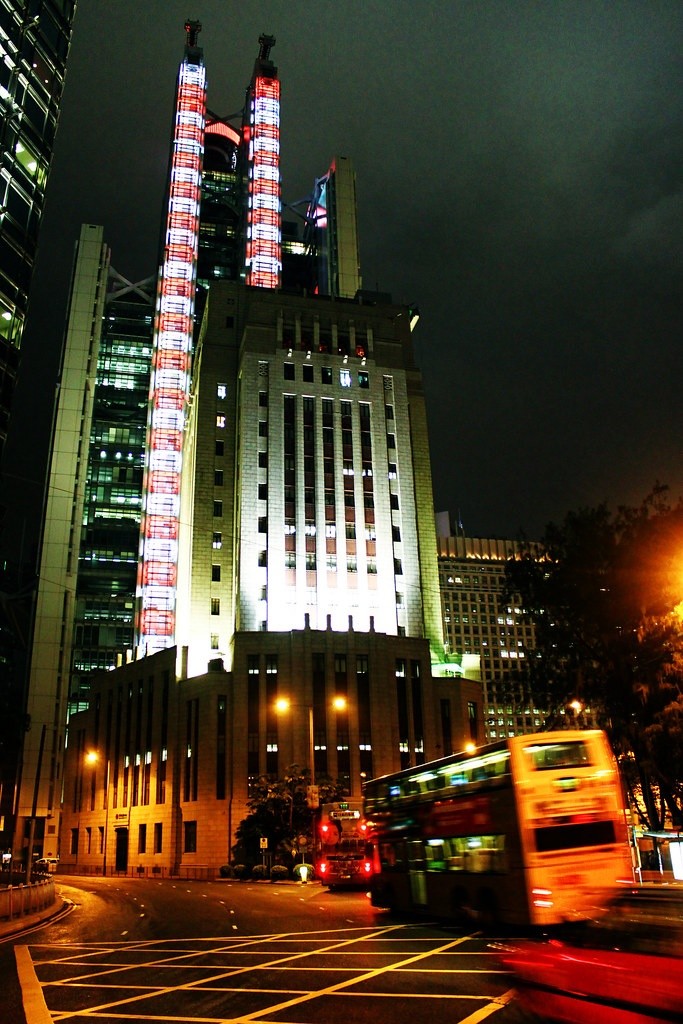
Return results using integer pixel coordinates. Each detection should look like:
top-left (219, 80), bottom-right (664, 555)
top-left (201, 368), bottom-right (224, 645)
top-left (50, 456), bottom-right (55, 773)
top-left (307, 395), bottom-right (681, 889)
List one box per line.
top-left (272, 692), bottom-right (353, 849)
top-left (85, 750), bottom-right (110, 877)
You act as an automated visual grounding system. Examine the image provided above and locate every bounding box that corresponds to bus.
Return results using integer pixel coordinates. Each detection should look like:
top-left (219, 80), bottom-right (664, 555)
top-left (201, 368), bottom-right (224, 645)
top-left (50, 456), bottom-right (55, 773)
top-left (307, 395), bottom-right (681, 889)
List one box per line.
top-left (362, 729), bottom-right (635, 939)
top-left (318, 802), bottom-right (381, 890)
